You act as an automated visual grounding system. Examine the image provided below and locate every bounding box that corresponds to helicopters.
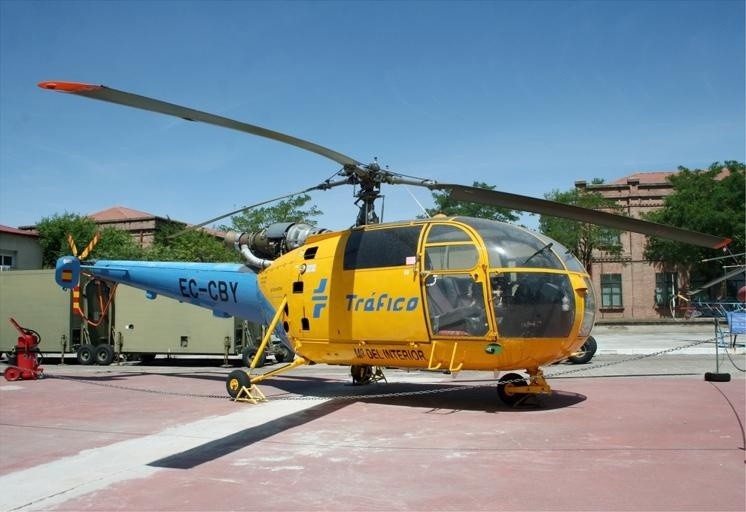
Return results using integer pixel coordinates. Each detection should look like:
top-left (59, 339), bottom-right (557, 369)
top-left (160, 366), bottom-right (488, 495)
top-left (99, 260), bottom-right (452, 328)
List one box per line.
top-left (36, 78), bottom-right (734, 401)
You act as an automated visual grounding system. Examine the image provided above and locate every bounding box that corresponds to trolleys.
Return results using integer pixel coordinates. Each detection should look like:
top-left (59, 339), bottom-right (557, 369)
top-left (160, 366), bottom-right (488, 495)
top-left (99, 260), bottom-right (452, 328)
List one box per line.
top-left (1, 317), bottom-right (44, 381)
